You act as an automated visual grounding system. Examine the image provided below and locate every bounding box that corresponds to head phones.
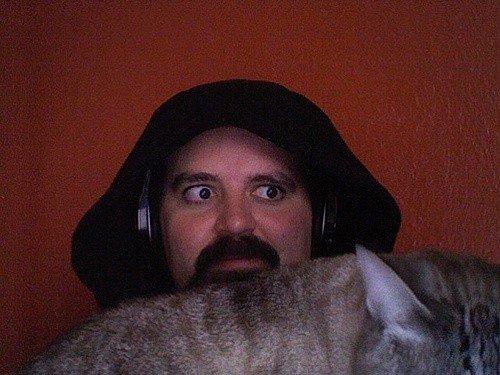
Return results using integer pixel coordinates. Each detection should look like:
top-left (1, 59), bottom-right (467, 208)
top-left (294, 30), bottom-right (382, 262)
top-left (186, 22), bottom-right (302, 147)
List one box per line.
top-left (134, 154), bottom-right (340, 262)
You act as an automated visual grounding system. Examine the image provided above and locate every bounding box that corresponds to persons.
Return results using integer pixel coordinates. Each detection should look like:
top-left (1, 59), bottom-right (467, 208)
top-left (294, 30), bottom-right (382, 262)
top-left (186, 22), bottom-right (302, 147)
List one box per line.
top-left (0, 79), bottom-right (500, 375)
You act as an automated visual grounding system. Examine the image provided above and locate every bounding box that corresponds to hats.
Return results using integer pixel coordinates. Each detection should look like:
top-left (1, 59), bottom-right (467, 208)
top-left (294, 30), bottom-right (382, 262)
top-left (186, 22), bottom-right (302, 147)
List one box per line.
top-left (72, 79), bottom-right (401, 308)
